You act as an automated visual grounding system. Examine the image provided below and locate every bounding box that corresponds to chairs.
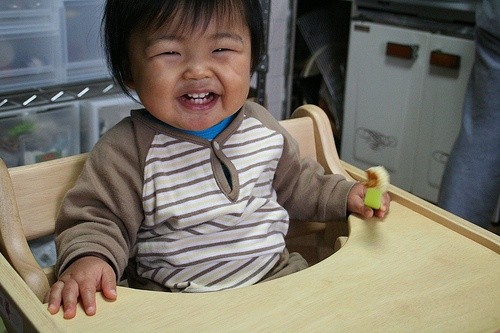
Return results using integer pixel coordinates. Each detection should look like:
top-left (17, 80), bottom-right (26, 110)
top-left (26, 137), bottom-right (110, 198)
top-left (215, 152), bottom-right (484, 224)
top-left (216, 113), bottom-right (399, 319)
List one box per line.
top-left (0, 105), bottom-right (500, 332)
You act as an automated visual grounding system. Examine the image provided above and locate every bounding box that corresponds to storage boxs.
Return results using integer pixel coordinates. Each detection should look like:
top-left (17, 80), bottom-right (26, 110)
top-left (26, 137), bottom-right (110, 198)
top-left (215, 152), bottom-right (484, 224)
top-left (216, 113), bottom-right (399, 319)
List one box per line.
top-left (1, 0), bottom-right (146, 167)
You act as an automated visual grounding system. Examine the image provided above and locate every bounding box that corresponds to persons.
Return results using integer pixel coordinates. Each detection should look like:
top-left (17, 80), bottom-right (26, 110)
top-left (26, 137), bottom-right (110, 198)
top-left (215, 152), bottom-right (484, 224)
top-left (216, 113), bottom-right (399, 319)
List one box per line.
top-left (436, 1), bottom-right (499, 229)
top-left (43, 0), bottom-right (390, 319)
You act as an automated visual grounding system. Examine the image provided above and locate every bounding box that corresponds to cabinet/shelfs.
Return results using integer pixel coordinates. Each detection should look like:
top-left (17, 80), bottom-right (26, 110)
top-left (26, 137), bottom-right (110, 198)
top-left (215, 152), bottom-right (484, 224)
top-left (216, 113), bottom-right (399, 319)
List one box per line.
top-left (340, 0), bottom-right (477, 207)
